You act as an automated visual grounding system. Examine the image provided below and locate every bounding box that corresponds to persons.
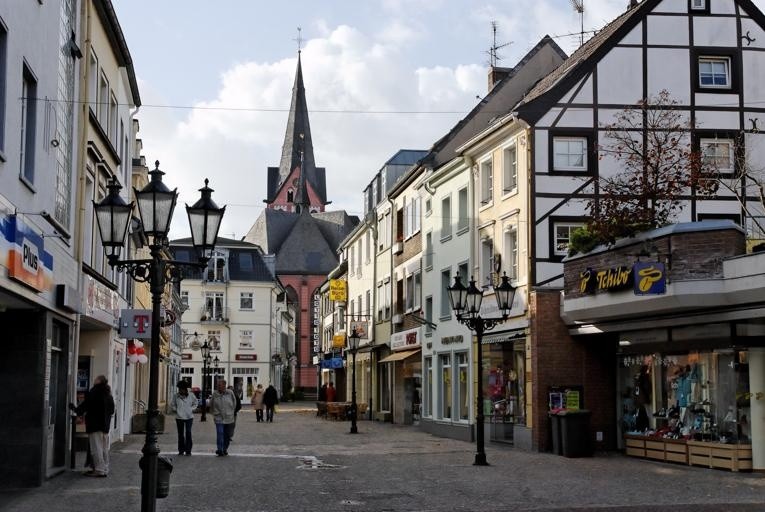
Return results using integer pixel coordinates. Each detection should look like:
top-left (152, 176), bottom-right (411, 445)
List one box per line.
top-left (171, 380), bottom-right (199, 456)
top-left (210, 379), bottom-right (242, 457)
top-left (264, 385), bottom-right (279, 423)
top-left (251, 384), bottom-right (264, 422)
top-left (69, 375), bottom-right (115, 478)
top-left (318, 382), bottom-right (336, 401)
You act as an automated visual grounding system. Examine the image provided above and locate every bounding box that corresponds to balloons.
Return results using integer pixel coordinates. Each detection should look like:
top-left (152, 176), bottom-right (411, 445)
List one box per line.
top-left (128, 341), bottom-right (148, 364)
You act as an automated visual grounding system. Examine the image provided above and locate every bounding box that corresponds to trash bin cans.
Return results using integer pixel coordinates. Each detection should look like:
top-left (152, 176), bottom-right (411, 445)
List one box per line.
top-left (557, 411), bottom-right (595, 458)
top-left (548, 412), bottom-right (578, 456)
top-left (139, 455), bottom-right (173, 498)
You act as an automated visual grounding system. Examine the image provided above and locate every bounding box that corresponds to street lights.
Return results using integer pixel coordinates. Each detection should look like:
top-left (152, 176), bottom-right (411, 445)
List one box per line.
top-left (445, 269), bottom-right (519, 466)
top-left (347, 325), bottom-right (363, 434)
top-left (88, 159), bottom-right (227, 512)
top-left (200, 338), bottom-right (220, 421)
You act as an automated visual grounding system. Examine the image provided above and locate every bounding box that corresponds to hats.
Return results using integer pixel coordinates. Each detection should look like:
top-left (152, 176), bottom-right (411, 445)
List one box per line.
top-left (177, 380), bottom-right (189, 388)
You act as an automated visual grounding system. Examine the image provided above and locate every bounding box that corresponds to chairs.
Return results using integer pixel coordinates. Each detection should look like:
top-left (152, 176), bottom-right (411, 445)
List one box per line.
top-left (315, 400), bottom-right (368, 422)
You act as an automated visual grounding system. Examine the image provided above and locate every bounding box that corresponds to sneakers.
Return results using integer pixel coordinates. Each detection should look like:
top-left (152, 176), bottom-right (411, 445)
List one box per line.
top-left (178, 452), bottom-right (191, 456)
top-left (84, 471), bottom-right (109, 477)
top-left (216, 450), bottom-right (228, 456)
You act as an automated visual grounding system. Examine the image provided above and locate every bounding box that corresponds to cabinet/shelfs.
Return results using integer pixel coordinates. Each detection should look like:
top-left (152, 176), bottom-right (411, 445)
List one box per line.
top-left (625, 429), bottom-right (752, 471)
